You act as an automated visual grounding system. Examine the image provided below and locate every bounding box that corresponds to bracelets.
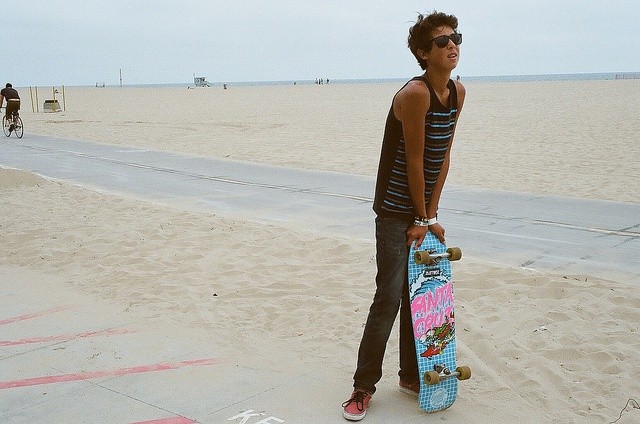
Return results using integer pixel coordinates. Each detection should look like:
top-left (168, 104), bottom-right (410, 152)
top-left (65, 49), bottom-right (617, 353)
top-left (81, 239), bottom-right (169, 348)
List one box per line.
top-left (428, 214), bottom-right (439, 226)
top-left (412, 216), bottom-right (429, 227)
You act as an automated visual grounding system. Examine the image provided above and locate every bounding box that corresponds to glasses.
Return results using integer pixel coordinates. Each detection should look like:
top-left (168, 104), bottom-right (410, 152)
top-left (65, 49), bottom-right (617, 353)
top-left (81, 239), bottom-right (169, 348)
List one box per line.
top-left (428, 34), bottom-right (462, 48)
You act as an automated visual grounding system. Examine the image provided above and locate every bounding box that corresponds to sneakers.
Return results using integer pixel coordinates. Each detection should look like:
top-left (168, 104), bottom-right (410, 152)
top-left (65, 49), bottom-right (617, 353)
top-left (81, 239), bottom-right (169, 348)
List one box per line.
top-left (399, 379), bottom-right (420, 397)
top-left (342, 388), bottom-right (374, 421)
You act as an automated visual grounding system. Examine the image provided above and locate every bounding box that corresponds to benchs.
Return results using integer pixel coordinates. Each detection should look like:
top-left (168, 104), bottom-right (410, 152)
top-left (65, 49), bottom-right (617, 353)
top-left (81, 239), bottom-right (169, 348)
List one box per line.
top-left (43, 99), bottom-right (63, 113)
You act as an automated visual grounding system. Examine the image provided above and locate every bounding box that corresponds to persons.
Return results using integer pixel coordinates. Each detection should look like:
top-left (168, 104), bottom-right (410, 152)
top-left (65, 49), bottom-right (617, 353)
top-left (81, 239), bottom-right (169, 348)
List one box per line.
top-left (0, 83), bottom-right (20, 132)
top-left (341, 9), bottom-right (466, 422)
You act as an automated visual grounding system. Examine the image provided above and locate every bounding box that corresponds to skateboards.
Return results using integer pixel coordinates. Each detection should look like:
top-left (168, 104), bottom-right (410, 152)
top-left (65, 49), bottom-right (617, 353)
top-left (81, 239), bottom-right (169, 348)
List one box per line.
top-left (408, 229), bottom-right (471, 414)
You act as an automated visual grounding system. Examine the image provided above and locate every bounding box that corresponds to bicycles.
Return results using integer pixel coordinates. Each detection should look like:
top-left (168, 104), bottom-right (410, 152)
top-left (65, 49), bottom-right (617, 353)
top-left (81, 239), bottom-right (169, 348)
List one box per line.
top-left (0, 105), bottom-right (24, 138)
